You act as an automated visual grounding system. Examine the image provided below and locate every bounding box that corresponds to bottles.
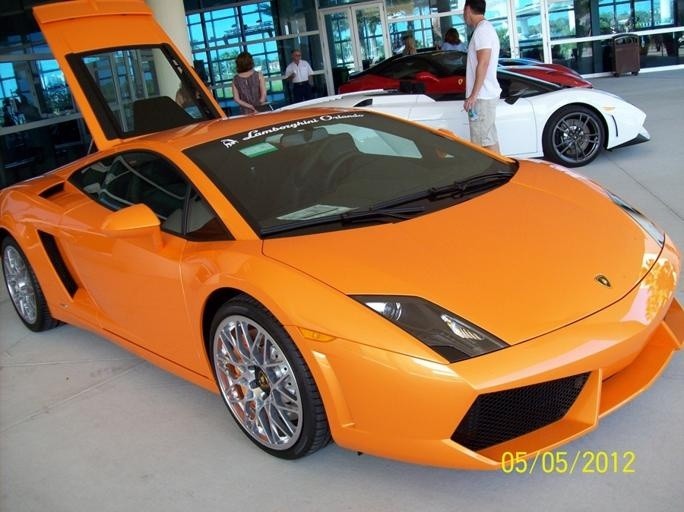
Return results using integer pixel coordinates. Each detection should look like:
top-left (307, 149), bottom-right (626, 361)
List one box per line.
top-left (468, 106), bottom-right (479, 121)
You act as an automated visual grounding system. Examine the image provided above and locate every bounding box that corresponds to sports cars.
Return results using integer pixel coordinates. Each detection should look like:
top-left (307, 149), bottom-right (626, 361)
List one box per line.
top-left (0, 0), bottom-right (683, 470)
top-left (270, 68), bottom-right (651, 175)
top-left (339, 47), bottom-right (591, 102)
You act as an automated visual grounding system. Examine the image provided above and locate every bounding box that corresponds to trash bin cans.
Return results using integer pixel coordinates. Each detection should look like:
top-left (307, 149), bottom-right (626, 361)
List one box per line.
top-left (612, 34), bottom-right (640, 77)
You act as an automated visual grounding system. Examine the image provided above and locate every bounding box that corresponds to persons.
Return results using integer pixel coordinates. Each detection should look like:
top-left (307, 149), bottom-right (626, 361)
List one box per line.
top-left (281, 49), bottom-right (315, 103)
top-left (464, 0), bottom-right (502, 160)
top-left (400, 33), bottom-right (415, 56)
top-left (175, 67), bottom-right (203, 107)
top-left (232, 52), bottom-right (267, 114)
top-left (435, 27), bottom-right (468, 54)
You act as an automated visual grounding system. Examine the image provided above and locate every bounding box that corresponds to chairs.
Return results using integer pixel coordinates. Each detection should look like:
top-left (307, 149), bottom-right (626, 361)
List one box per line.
top-left (125, 164), bottom-right (194, 222)
